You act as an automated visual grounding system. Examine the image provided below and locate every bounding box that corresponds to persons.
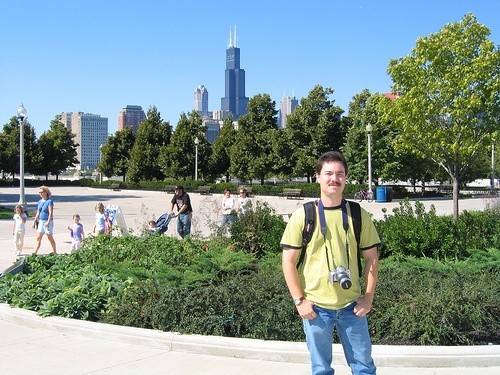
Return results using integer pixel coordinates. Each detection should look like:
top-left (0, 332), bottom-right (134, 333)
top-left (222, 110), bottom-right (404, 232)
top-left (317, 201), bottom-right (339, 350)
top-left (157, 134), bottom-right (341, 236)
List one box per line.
top-left (92, 202), bottom-right (107, 235)
top-left (12, 205), bottom-right (28, 256)
top-left (148, 221), bottom-right (157, 229)
top-left (236, 188), bottom-right (252, 219)
top-left (280, 151), bottom-right (381, 375)
top-left (219, 188), bottom-right (235, 227)
top-left (104, 211), bottom-right (112, 235)
top-left (171, 185), bottom-right (193, 239)
top-left (32, 186), bottom-right (56, 255)
top-left (68, 214), bottom-right (84, 251)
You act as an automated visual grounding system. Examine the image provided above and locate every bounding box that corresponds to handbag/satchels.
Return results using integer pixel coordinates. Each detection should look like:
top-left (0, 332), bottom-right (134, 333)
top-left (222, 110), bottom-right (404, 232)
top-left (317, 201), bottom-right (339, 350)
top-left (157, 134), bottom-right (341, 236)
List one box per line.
top-left (71, 231), bottom-right (73, 237)
top-left (35, 222), bottom-right (38, 230)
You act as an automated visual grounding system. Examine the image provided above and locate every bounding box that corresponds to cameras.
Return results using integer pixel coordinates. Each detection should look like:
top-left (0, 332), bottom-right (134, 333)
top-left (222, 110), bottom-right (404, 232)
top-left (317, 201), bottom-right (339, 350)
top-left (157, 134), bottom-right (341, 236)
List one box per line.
top-left (330, 266), bottom-right (352, 290)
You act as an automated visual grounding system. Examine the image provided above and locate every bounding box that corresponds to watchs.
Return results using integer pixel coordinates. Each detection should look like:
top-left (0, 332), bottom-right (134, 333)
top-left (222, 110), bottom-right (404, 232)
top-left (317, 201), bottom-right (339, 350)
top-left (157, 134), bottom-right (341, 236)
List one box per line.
top-left (294, 297), bottom-right (305, 306)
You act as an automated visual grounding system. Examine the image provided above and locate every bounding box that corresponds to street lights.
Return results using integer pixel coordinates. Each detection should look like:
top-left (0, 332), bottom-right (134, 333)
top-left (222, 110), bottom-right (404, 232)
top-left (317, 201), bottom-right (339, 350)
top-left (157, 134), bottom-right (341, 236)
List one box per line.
top-left (194, 137), bottom-right (199, 182)
top-left (16, 103), bottom-right (28, 210)
top-left (366, 122), bottom-right (374, 194)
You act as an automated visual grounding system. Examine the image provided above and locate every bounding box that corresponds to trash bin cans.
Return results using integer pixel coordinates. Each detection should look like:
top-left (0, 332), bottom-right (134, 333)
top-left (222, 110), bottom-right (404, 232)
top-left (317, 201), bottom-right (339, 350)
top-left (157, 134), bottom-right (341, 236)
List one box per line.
top-left (376, 187), bottom-right (387, 203)
top-left (387, 187), bottom-right (393, 202)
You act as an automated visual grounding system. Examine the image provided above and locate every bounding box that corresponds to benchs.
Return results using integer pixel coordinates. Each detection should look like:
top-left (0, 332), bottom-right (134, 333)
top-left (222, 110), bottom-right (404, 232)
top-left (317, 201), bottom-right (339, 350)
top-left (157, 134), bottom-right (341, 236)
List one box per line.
top-left (233, 187), bottom-right (253, 197)
top-left (161, 185), bottom-right (175, 194)
top-left (194, 186), bottom-right (212, 195)
top-left (108, 184), bottom-right (120, 191)
top-left (279, 188), bottom-right (301, 199)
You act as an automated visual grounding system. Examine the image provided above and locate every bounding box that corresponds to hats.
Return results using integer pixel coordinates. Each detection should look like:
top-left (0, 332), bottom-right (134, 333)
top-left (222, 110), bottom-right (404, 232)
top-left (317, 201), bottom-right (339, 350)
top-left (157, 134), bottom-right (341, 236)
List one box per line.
top-left (239, 188), bottom-right (247, 193)
top-left (173, 186), bottom-right (183, 189)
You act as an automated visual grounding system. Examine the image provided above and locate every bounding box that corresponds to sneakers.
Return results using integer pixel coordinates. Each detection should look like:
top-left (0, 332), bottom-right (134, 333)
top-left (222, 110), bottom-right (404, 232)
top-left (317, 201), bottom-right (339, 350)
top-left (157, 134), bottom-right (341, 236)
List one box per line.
top-left (16, 250), bottom-right (20, 256)
top-left (14, 250), bottom-right (18, 254)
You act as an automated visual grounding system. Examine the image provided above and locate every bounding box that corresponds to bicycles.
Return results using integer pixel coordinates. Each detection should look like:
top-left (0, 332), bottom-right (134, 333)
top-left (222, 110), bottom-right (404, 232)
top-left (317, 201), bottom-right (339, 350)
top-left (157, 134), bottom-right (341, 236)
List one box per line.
top-left (354, 188), bottom-right (374, 203)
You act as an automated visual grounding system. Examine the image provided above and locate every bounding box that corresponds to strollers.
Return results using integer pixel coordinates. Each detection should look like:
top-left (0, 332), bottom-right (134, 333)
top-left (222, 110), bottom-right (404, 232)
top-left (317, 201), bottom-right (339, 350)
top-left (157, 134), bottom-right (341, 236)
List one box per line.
top-left (139, 213), bottom-right (173, 238)
